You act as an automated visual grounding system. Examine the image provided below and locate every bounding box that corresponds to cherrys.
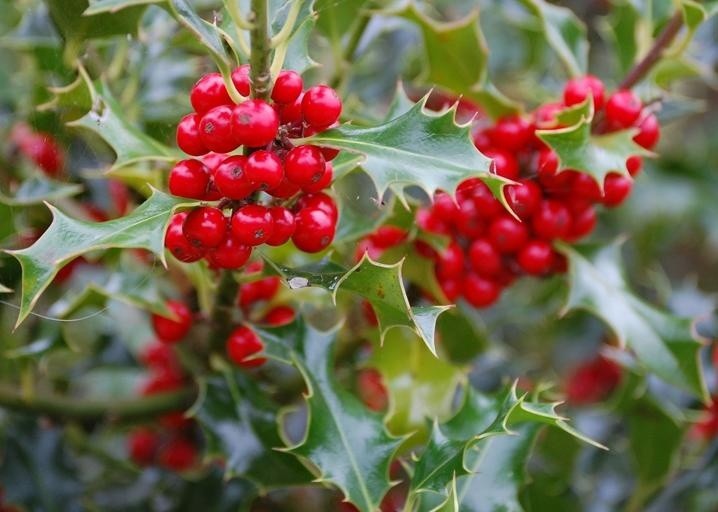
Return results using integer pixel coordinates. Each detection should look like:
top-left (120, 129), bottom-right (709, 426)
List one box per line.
top-left (4, 65), bottom-right (718, 511)
